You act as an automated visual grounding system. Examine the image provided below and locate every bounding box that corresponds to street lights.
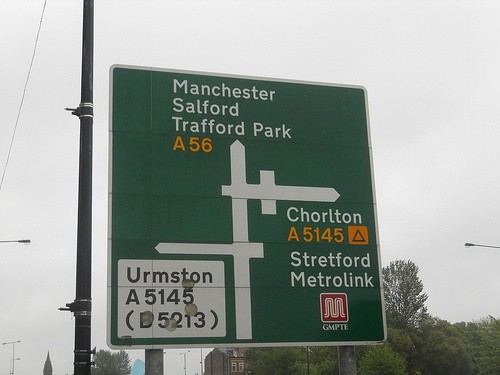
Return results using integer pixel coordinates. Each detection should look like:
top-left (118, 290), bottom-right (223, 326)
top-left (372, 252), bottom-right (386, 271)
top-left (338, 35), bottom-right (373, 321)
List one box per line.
top-left (2, 341), bottom-right (21, 375)
top-left (10, 358), bottom-right (21, 375)
